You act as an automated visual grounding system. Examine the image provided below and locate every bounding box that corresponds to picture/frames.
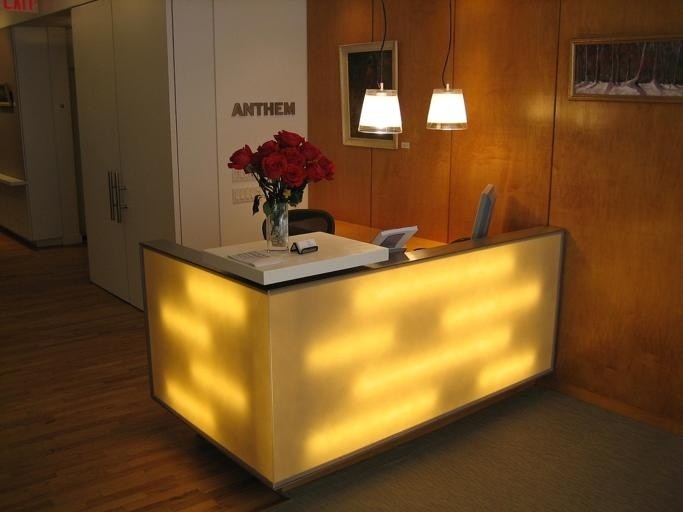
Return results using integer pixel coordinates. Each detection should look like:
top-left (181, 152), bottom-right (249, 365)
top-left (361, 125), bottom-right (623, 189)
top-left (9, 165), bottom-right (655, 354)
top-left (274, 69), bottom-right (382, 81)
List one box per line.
top-left (567, 33), bottom-right (683, 104)
top-left (338, 40), bottom-right (398, 149)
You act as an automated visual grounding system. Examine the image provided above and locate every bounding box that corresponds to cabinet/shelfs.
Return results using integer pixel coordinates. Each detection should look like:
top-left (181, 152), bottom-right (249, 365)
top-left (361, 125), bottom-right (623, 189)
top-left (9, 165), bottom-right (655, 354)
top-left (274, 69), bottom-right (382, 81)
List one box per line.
top-left (70, 0), bottom-right (308, 312)
top-left (0, 25), bottom-right (85, 249)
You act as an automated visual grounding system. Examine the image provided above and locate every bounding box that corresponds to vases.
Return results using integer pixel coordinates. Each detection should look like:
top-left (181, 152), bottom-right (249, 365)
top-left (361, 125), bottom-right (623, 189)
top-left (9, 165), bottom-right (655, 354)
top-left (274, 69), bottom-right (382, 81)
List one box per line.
top-left (265, 199), bottom-right (290, 254)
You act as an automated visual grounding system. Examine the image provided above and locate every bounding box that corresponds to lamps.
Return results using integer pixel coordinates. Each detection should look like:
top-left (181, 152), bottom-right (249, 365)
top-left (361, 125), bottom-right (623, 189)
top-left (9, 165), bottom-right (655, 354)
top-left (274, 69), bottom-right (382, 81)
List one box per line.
top-left (426, 0), bottom-right (469, 131)
top-left (357, 0), bottom-right (402, 135)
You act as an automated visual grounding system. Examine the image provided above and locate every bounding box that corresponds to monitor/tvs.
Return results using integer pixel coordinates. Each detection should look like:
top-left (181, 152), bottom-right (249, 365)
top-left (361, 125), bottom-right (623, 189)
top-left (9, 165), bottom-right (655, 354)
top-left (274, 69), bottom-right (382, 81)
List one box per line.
top-left (470, 184), bottom-right (496, 239)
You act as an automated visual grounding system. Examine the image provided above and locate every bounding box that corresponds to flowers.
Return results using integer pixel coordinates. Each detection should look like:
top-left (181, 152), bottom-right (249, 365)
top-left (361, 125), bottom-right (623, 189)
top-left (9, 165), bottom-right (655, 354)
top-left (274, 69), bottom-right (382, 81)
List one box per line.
top-left (227, 129), bottom-right (337, 190)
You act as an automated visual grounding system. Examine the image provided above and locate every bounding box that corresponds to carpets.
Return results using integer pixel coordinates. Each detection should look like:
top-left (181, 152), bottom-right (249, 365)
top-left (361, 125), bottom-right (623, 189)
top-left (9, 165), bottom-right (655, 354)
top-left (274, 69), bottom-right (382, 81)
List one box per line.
top-left (258, 385), bottom-right (683, 512)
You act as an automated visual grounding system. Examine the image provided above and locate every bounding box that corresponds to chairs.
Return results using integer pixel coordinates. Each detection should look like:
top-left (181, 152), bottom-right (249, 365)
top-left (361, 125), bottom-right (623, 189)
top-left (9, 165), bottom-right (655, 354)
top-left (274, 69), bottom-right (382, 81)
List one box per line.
top-left (261, 208), bottom-right (335, 239)
